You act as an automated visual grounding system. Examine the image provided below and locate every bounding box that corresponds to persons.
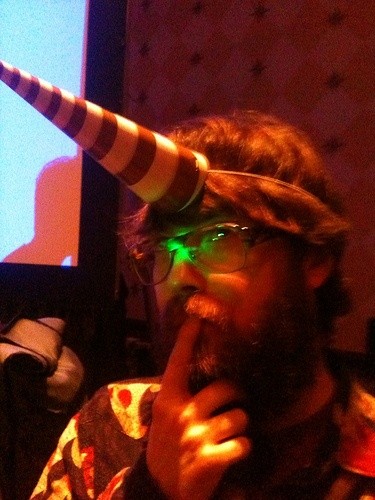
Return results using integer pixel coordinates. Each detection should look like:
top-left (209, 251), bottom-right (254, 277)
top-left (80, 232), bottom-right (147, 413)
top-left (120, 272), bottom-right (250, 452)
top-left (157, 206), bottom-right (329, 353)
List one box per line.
top-left (26, 108), bottom-right (373, 499)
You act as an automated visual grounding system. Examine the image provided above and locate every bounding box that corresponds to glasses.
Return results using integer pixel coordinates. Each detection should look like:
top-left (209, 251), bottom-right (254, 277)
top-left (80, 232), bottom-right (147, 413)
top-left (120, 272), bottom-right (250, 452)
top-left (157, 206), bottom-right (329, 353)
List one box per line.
top-left (125, 221), bottom-right (289, 288)
